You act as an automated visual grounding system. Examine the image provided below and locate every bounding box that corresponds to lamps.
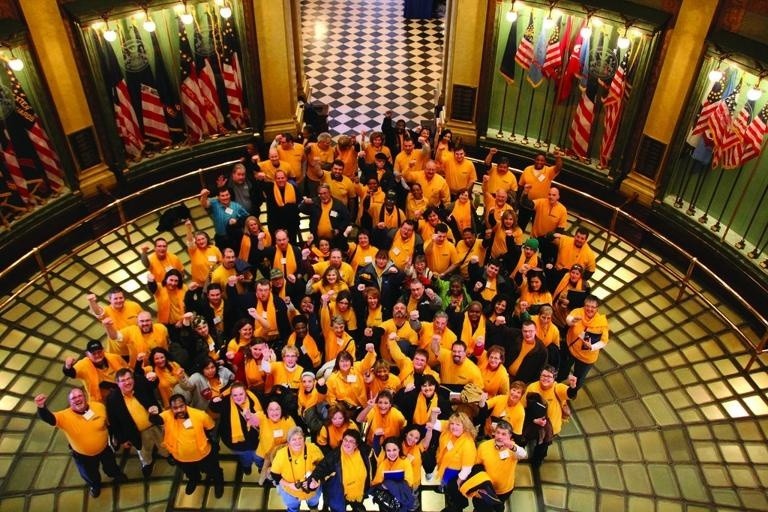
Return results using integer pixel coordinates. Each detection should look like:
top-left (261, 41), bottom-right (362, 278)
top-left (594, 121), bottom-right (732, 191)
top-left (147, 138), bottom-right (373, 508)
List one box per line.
top-left (505, 1), bottom-right (640, 51)
top-left (96, 0), bottom-right (233, 42)
top-left (706, 43), bottom-right (768, 102)
top-left (0, 40), bottom-right (27, 71)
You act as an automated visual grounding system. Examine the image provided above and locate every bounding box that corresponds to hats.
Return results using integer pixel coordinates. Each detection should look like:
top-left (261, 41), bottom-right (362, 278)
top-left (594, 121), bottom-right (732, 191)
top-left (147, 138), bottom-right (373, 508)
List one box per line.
top-left (86, 339), bottom-right (104, 353)
top-left (525, 237), bottom-right (540, 252)
top-left (270, 267), bottom-right (284, 280)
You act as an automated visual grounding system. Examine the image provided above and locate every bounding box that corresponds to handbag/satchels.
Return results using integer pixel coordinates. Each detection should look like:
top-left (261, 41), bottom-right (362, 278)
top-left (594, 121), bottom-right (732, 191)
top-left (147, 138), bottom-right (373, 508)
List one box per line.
top-left (302, 402), bottom-right (329, 432)
top-left (374, 489), bottom-right (401, 512)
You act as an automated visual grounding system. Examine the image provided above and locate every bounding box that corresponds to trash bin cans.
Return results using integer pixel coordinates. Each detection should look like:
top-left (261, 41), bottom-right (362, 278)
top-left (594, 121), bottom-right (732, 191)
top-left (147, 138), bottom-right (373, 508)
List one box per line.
top-left (309, 101), bottom-right (328, 132)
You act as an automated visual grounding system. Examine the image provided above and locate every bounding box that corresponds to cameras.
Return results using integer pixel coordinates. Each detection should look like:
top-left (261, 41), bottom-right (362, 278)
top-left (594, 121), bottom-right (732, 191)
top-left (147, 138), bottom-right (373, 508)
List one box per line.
top-left (396, 127), bottom-right (404, 134)
top-left (295, 480), bottom-right (303, 489)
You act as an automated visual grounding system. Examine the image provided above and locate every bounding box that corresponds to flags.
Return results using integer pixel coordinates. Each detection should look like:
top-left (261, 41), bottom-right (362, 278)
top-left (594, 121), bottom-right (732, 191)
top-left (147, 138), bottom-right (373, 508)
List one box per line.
top-left (90, 0), bottom-right (249, 163)
top-left (1, 68), bottom-right (66, 203)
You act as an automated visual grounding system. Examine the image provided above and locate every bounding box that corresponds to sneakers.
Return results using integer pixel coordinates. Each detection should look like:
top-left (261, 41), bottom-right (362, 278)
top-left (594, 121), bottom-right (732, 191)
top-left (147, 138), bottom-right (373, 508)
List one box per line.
top-left (166, 454), bottom-right (177, 466)
top-left (213, 466), bottom-right (223, 498)
top-left (241, 464), bottom-right (251, 475)
top-left (186, 473), bottom-right (202, 496)
top-left (114, 473), bottom-right (127, 484)
top-left (89, 483), bottom-right (99, 497)
top-left (141, 460), bottom-right (153, 477)
